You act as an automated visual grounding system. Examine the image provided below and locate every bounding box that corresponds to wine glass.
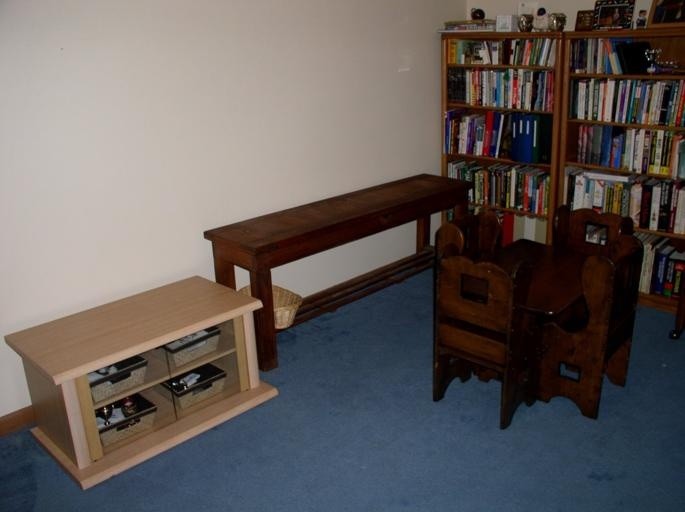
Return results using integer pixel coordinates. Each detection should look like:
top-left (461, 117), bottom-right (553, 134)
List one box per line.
top-left (98, 407), bottom-right (114, 425)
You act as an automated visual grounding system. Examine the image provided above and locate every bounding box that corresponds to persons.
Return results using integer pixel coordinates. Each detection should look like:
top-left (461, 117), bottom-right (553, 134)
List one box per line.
top-left (611, 7), bottom-right (624, 25)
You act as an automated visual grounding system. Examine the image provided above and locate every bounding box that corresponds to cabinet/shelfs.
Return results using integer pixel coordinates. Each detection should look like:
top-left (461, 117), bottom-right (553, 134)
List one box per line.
top-left (442, 33), bottom-right (564, 245)
top-left (4, 276), bottom-right (279, 492)
top-left (557, 28), bottom-right (685, 339)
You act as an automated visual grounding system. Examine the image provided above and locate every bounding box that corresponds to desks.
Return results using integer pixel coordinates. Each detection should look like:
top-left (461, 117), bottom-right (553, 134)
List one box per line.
top-left (462, 239), bottom-right (589, 329)
top-left (204, 174), bottom-right (475, 371)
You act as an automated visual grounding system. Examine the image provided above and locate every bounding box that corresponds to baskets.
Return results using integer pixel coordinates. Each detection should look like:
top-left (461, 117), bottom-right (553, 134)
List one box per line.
top-left (237, 283), bottom-right (304, 330)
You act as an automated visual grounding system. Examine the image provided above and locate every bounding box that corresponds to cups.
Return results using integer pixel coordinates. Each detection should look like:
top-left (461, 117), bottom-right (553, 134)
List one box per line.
top-left (518, 14), bottom-right (534, 31)
top-left (548, 13), bottom-right (566, 31)
top-left (121, 398), bottom-right (139, 418)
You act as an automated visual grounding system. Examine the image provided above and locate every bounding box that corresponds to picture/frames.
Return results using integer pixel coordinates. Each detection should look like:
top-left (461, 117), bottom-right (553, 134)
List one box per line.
top-left (592, 0), bottom-right (635, 30)
top-left (646, 0), bottom-right (685, 28)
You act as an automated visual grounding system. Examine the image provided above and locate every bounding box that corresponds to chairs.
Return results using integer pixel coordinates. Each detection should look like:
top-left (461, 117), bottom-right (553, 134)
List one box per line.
top-left (433, 216), bottom-right (496, 383)
top-left (552, 206), bottom-right (633, 255)
top-left (538, 234), bottom-right (644, 420)
top-left (433, 256), bottom-right (539, 429)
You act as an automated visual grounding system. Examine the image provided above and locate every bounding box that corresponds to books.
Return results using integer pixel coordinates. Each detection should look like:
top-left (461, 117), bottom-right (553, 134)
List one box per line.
top-left (444, 38), bottom-right (556, 246)
top-left (564, 35), bottom-right (684, 299)
